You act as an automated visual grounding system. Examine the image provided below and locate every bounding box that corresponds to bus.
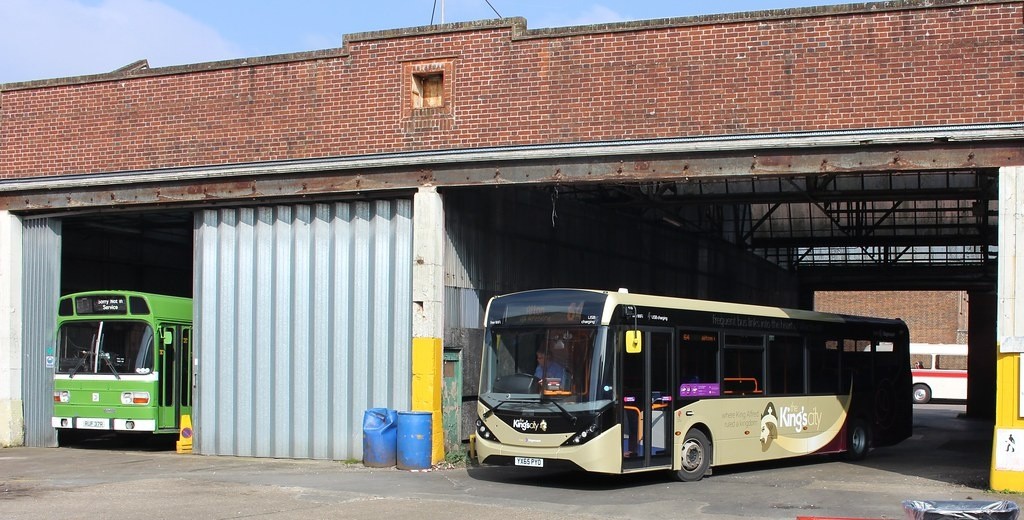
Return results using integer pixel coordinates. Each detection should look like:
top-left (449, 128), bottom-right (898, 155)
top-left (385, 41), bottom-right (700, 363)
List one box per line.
top-left (50, 289), bottom-right (194, 451)
top-left (863, 342), bottom-right (968, 405)
top-left (470, 286), bottom-right (914, 484)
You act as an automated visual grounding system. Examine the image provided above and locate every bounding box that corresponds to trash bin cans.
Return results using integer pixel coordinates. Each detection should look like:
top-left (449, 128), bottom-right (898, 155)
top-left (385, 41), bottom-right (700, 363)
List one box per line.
top-left (396, 409), bottom-right (433, 472)
top-left (361, 408), bottom-right (398, 469)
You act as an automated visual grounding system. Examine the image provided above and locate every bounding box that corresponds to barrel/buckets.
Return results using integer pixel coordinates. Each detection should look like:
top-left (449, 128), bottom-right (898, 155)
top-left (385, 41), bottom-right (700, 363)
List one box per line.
top-left (363, 408), bottom-right (397, 467)
top-left (397, 412), bottom-right (433, 470)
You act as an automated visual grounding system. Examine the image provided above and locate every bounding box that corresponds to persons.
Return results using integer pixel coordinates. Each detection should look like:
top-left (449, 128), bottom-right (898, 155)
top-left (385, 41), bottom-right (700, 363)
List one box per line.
top-left (534, 349), bottom-right (569, 391)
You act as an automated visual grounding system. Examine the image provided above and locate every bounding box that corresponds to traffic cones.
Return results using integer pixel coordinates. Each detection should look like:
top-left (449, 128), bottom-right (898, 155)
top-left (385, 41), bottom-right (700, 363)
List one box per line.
top-left (176, 414), bottom-right (194, 454)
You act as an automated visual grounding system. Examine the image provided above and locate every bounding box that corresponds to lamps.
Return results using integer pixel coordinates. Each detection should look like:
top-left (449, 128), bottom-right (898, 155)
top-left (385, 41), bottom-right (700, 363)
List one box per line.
top-left (661, 215), bottom-right (681, 227)
